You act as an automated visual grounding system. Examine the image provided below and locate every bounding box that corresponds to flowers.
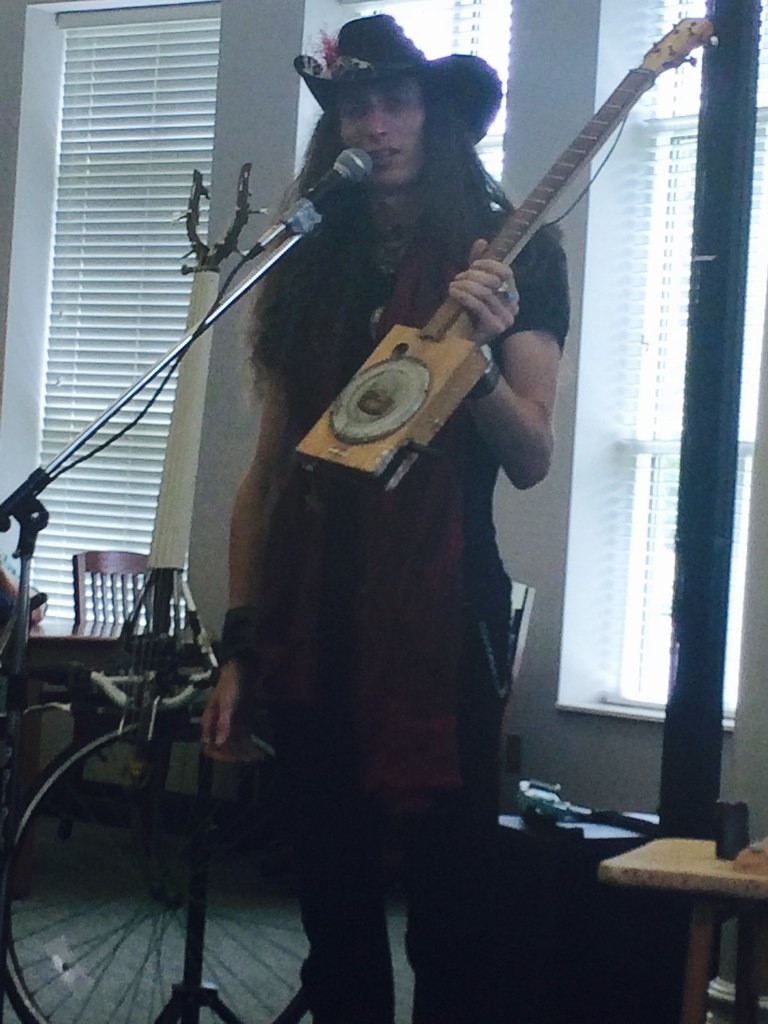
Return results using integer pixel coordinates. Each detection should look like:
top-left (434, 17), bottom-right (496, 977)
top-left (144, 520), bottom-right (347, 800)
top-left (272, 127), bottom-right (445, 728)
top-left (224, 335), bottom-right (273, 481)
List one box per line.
top-left (314, 28), bottom-right (339, 67)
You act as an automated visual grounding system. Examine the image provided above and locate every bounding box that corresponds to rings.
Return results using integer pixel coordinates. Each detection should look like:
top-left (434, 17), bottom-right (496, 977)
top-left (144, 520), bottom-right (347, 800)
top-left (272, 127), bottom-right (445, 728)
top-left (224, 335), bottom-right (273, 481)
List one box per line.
top-left (498, 282), bottom-right (511, 300)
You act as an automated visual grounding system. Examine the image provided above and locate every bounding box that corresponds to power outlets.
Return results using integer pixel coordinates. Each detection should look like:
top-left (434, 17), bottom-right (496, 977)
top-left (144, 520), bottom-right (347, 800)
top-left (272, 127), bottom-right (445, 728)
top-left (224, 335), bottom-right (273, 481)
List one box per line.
top-left (504, 734), bottom-right (522, 776)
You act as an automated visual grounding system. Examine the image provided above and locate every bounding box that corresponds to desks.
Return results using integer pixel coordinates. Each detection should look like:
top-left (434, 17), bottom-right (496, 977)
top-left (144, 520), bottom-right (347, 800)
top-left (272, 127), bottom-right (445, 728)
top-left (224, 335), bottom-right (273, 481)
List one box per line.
top-left (598, 835), bottom-right (768, 1024)
top-left (0, 637), bottom-right (225, 900)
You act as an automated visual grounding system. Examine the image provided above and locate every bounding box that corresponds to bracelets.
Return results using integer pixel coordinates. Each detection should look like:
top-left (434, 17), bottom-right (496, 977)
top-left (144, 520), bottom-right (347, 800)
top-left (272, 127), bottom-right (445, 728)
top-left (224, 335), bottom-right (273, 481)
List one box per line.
top-left (462, 362), bottom-right (502, 401)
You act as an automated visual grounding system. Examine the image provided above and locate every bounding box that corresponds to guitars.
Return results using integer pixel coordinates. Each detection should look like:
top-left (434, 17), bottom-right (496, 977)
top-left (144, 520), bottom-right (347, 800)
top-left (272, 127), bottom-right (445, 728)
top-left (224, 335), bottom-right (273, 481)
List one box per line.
top-left (295, 17), bottom-right (717, 492)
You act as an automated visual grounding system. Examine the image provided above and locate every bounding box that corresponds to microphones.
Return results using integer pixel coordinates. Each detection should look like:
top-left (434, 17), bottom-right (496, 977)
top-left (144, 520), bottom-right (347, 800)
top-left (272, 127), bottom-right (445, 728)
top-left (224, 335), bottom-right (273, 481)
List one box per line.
top-left (247, 147), bottom-right (373, 259)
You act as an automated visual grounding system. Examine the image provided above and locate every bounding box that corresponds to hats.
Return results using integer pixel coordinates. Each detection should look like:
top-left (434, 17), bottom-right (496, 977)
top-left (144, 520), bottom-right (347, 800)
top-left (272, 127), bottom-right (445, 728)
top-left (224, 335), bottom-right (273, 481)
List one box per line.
top-left (293, 14), bottom-right (503, 147)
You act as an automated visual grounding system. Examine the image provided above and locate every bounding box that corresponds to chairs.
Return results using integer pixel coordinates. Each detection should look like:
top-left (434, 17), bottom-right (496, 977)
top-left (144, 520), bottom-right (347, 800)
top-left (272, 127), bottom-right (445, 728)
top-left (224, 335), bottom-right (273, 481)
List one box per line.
top-left (500, 579), bottom-right (537, 733)
top-left (58, 550), bottom-right (210, 839)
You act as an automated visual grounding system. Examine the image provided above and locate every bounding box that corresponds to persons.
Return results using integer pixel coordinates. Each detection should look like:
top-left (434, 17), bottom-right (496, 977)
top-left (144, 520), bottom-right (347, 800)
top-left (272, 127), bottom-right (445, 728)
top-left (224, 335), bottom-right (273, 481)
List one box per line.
top-left (203, 13), bottom-right (572, 1024)
